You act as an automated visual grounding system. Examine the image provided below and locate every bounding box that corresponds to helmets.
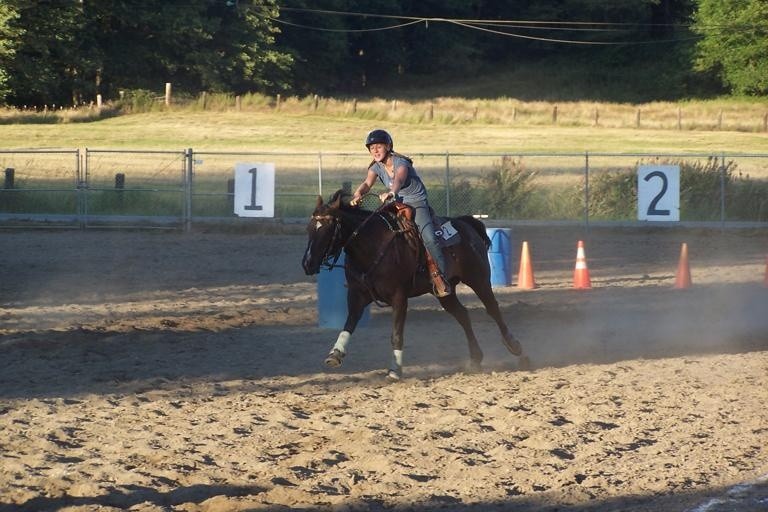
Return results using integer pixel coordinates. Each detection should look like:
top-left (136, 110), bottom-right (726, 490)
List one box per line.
top-left (365, 129), bottom-right (393, 150)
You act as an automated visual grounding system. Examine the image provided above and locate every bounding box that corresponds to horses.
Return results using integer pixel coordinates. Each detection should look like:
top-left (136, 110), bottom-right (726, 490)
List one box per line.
top-left (301, 188), bottom-right (533, 382)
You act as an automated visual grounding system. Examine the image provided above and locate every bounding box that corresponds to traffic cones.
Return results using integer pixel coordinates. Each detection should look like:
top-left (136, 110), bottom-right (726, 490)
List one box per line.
top-left (568, 240), bottom-right (593, 290)
top-left (514, 240), bottom-right (539, 290)
top-left (673, 242), bottom-right (693, 290)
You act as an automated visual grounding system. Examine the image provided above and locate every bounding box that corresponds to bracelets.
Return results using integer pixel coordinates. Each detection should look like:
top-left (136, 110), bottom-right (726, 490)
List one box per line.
top-left (390, 192), bottom-right (396, 199)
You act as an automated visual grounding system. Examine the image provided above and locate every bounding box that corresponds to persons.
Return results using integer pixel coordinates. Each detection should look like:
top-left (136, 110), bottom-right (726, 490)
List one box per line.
top-left (350, 130), bottom-right (451, 297)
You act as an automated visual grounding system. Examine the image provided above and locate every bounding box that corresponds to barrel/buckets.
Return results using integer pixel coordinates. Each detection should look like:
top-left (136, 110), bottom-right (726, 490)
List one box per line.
top-left (486, 228), bottom-right (512, 289)
top-left (317, 249), bottom-right (369, 329)
